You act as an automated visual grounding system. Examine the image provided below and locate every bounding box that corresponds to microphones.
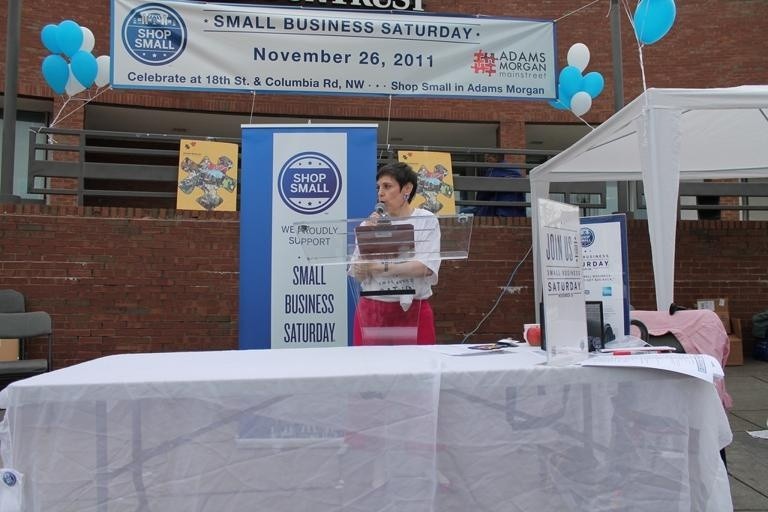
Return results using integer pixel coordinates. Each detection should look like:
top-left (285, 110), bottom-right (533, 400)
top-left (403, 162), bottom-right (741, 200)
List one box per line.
top-left (375, 202), bottom-right (386, 214)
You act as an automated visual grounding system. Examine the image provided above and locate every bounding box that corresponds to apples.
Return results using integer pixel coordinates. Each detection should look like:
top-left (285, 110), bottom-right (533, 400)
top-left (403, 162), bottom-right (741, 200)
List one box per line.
top-left (527, 325), bottom-right (541, 346)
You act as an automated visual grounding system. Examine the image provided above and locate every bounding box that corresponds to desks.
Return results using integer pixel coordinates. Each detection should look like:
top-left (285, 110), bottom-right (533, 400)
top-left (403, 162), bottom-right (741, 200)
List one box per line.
top-left (0, 342), bottom-right (737, 512)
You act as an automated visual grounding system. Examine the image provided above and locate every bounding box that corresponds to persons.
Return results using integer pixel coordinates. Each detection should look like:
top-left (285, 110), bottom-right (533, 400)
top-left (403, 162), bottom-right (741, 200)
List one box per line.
top-left (348, 162), bottom-right (442, 345)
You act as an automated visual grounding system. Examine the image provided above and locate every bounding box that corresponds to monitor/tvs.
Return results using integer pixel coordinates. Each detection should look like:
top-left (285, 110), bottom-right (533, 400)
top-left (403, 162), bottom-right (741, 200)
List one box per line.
top-left (539, 300), bottom-right (605, 351)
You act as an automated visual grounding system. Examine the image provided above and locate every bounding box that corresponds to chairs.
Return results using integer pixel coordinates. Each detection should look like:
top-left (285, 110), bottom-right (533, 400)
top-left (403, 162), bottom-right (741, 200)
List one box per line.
top-left (0, 310), bottom-right (53, 374)
top-left (0, 289), bottom-right (26, 361)
top-left (628, 309), bottom-right (730, 468)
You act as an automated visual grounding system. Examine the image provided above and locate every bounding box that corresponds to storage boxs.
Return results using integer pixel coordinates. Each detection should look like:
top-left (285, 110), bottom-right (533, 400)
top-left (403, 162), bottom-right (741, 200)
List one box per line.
top-left (695, 297), bottom-right (743, 366)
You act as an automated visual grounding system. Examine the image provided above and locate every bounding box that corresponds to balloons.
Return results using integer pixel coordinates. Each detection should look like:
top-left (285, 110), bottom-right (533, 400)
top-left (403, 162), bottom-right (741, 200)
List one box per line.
top-left (40, 25), bottom-right (63, 54)
top-left (546, 43), bottom-right (605, 120)
top-left (633, 0), bottom-right (677, 46)
top-left (65, 62), bottom-right (86, 98)
top-left (71, 51), bottom-right (98, 90)
top-left (55, 20), bottom-right (84, 58)
top-left (80, 25), bottom-right (95, 55)
top-left (42, 55), bottom-right (70, 96)
top-left (94, 55), bottom-right (110, 90)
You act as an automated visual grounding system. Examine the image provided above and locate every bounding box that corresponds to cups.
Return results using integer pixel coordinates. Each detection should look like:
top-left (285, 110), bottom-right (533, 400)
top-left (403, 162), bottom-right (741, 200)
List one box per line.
top-left (522, 322), bottom-right (543, 346)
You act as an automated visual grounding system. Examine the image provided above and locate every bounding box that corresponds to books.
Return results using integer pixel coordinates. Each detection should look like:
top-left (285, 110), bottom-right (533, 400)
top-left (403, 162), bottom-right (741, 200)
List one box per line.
top-left (355, 223), bottom-right (418, 297)
